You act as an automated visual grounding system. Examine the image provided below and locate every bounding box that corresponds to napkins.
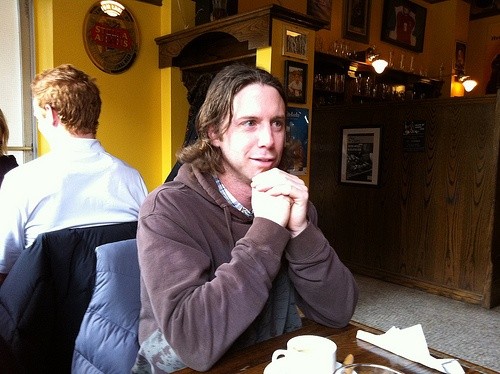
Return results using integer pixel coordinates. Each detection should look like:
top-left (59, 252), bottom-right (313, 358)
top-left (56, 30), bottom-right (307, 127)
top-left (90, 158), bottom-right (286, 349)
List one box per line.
top-left (356, 323), bottom-right (465, 374)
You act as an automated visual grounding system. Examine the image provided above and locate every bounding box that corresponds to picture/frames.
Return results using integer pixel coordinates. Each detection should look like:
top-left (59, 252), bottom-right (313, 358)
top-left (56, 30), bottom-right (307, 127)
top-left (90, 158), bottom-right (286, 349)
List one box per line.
top-left (285, 60), bottom-right (308, 103)
top-left (338, 125), bottom-right (384, 188)
top-left (282, 25), bottom-right (309, 60)
top-left (342, 0), bottom-right (372, 44)
top-left (381, 0), bottom-right (427, 53)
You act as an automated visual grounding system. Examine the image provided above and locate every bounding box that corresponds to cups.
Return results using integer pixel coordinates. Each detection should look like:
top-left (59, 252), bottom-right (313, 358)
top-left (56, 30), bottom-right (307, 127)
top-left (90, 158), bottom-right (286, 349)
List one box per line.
top-left (315, 37), bottom-right (427, 100)
top-left (272, 335), bottom-right (337, 374)
top-left (334, 362), bottom-right (405, 373)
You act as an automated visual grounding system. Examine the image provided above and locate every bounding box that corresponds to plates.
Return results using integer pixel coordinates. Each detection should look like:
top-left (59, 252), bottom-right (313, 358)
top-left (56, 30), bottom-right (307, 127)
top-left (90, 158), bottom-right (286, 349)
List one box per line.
top-left (263, 357), bottom-right (357, 374)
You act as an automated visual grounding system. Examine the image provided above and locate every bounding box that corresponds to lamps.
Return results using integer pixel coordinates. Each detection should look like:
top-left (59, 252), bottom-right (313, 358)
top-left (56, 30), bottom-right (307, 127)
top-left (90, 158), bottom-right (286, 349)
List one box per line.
top-left (366, 48), bottom-right (388, 74)
top-left (456, 71), bottom-right (477, 92)
top-left (101, 0), bottom-right (125, 17)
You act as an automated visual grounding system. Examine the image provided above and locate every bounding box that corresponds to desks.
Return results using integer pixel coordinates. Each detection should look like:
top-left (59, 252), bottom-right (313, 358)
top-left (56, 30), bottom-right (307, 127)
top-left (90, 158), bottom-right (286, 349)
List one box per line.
top-left (169, 320), bottom-right (500, 374)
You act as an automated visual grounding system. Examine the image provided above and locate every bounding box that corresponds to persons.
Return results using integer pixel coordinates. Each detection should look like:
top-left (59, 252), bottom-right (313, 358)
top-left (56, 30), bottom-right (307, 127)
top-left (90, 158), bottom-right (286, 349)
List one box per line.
top-left (0, 64), bottom-right (146, 282)
top-left (136, 65), bottom-right (359, 374)
top-left (289, 71), bottom-right (303, 98)
